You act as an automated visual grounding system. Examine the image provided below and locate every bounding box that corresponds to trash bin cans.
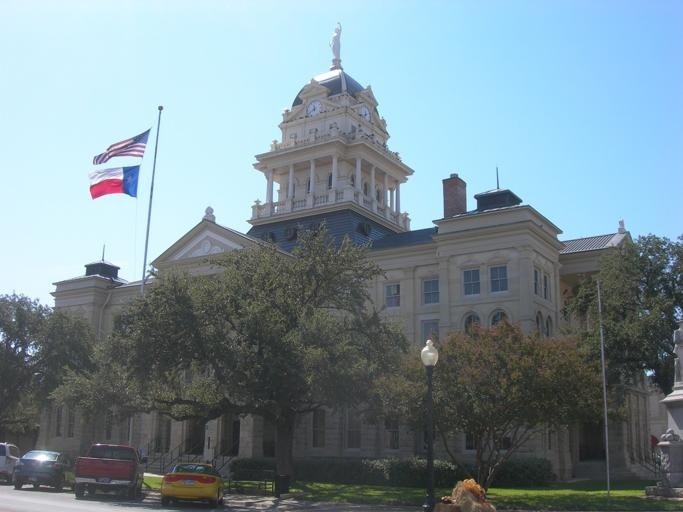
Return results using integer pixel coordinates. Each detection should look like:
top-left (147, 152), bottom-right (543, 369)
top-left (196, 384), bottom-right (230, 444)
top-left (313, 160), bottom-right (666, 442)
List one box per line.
top-left (280, 474), bottom-right (289, 494)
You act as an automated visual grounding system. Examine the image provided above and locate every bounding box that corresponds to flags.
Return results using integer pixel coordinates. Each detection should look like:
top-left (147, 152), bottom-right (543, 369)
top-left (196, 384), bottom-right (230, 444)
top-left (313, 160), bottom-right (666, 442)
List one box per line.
top-left (92, 127), bottom-right (151, 166)
top-left (88, 165), bottom-right (141, 200)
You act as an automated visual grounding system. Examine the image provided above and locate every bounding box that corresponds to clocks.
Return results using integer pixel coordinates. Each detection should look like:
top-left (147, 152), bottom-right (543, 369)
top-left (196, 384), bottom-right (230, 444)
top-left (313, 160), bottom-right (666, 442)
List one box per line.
top-left (306, 100), bottom-right (322, 117)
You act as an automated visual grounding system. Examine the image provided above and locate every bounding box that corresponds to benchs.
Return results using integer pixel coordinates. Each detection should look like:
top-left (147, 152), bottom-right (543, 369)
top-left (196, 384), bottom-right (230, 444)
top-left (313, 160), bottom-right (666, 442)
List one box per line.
top-left (229, 479), bottom-right (274, 496)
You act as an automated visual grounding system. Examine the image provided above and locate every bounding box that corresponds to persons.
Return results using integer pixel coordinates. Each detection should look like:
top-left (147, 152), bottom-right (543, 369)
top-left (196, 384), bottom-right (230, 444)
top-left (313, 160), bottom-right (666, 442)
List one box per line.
top-left (649, 434), bottom-right (658, 460)
top-left (327, 19), bottom-right (341, 60)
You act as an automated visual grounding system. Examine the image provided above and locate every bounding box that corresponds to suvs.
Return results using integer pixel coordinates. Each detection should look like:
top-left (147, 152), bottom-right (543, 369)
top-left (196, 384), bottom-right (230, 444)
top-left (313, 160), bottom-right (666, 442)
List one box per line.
top-left (0, 441), bottom-right (21, 484)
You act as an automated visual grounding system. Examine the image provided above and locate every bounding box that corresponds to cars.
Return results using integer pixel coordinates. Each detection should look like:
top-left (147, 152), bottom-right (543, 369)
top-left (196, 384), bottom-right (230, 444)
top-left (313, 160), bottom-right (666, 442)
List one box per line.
top-left (160, 461), bottom-right (224, 506)
top-left (14, 449), bottom-right (72, 493)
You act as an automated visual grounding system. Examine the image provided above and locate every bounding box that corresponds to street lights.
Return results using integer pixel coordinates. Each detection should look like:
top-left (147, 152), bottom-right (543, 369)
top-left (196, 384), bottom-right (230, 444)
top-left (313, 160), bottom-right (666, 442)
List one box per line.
top-left (421, 339), bottom-right (438, 509)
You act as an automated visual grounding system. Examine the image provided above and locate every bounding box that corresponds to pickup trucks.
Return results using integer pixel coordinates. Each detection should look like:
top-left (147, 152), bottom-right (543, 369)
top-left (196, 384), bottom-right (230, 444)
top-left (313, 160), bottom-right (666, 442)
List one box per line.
top-left (74, 443), bottom-right (147, 501)
